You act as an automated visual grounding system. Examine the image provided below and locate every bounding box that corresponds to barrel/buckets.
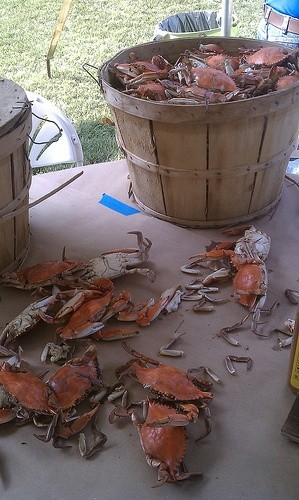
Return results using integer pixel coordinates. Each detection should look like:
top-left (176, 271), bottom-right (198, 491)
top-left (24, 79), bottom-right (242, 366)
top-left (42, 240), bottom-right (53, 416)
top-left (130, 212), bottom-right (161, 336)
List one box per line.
top-left (1, 77), bottom-right (62, 290)
top-left (81, 35), bottom-right (299, 229)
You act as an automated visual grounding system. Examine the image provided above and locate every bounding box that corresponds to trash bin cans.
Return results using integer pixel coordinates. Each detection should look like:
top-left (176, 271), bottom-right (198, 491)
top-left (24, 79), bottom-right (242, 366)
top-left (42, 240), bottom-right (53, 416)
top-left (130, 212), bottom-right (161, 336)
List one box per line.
top-left (155, 10), bottom-right (241, 40)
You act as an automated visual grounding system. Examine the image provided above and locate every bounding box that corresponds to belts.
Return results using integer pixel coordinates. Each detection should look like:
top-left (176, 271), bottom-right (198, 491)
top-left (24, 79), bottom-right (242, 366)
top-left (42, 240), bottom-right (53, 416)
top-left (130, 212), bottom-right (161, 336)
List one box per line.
top-left (264, 5), bottom-right (299, 34)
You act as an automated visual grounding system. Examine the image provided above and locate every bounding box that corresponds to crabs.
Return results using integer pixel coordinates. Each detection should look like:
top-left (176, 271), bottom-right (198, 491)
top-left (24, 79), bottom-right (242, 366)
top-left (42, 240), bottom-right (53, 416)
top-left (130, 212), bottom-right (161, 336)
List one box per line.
top-left (0, 222), bottom-right (299, 488)
top-left (112, 42), bottom-right (299, 106)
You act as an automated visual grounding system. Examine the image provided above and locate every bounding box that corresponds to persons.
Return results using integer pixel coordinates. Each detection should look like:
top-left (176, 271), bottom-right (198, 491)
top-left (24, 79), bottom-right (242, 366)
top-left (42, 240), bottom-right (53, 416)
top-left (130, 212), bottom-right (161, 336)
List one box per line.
top-left (255, 0), bottom-right (298, 47)
